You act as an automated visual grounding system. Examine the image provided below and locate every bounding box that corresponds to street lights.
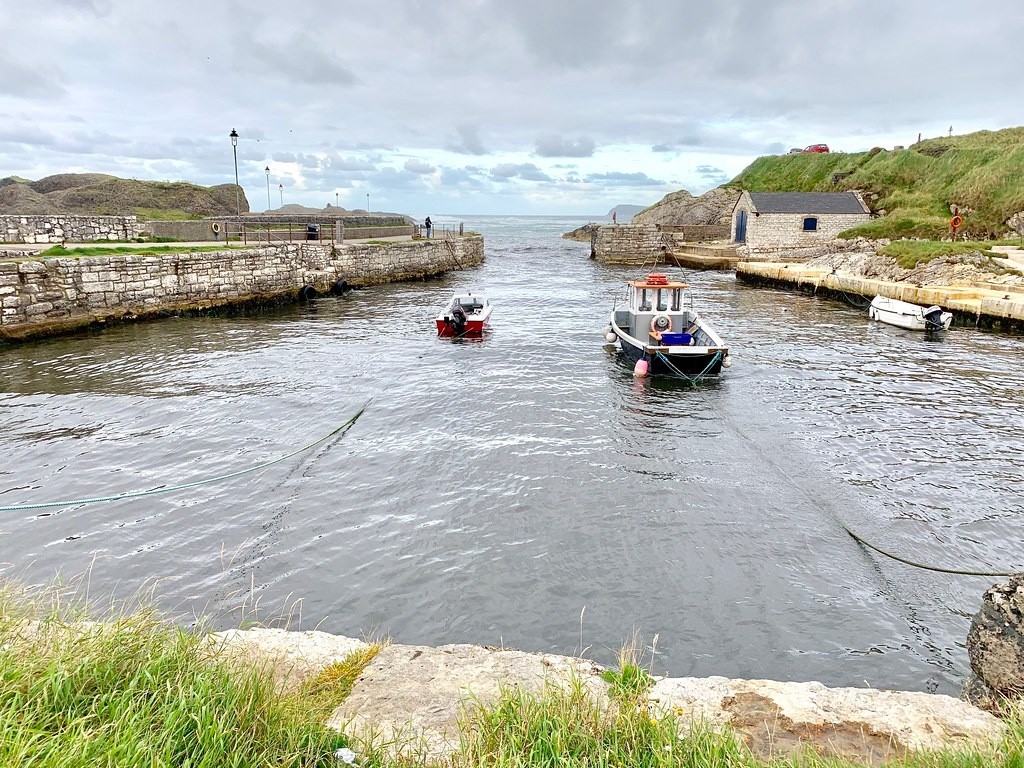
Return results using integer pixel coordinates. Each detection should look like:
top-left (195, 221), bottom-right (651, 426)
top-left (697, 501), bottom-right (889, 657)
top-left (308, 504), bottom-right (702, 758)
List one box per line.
top-left (336, 193), bottom-right (339, 207)
top-left (279, 184), bottom-right (283, 208)
top-left (264, 166), bottom-right (270, 212)
top-left (367, 193), bottom-right (369, 213)
top-left (230, 128), bottom-right (240, 225)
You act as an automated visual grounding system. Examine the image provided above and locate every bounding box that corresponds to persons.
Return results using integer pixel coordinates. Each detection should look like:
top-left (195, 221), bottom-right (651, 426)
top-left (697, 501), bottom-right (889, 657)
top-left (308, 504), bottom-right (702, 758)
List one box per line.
top-left (425, 216), bottom-right (432, 238)
top-left (613, 212), bottom-right (616, 223)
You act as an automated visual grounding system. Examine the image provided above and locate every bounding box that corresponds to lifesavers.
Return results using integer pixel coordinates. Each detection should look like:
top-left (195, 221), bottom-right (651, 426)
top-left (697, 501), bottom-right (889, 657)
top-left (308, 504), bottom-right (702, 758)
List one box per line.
top-left (644, 274), bottom-right (668, 284)
top-left (337, 280), bottom-right (347, 296)
top-left (950, 216), bottom-right (962, 228)
top-left (303, 286), bottom-right (314, 296)
top-left (211, 223), bottom-right (220, 233)
top-left (651, 315), bottom-right (672, 333)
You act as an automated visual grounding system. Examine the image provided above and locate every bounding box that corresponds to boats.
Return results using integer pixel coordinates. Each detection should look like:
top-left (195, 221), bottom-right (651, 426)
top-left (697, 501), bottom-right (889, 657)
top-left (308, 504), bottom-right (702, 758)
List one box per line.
top-left (609, 230), bottom-right (729, 381)
top-left (436, 291), bottom-right (495, 343)
top-left (868, 294), bottom-right (953, 332)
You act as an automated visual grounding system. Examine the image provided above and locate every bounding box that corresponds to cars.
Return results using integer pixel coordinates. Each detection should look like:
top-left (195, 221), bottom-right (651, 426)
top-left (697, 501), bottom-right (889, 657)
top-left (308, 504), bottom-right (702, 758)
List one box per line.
top-left (801, 143), bottom-right (829, 153)
top-left (787, 147), bottom-right (803, 155)
